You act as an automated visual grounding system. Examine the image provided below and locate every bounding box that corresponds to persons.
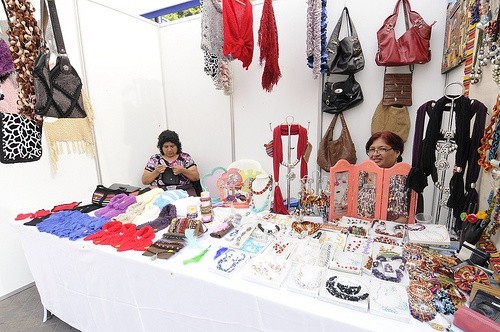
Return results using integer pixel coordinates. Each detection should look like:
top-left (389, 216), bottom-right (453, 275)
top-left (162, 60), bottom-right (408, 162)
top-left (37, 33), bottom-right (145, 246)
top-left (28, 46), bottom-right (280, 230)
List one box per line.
top-left (341, 130), bottom-right (409, 224)
top-left (142, 129), bottom-right (200, 197)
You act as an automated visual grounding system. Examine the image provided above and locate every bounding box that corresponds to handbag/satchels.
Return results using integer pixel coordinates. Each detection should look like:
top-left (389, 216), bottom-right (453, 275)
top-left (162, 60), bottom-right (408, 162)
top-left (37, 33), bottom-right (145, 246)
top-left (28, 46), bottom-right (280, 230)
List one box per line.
top-left (371, 98), bottom-right (411, 143)
top-left (382, 67), bottom-right (414, 106)
top-left (326, 7), bottom-right (365, 75)
top-left (92, 183), bottom-right (142, 207)
top-left (162, 167), bottom-right (181, 184)
top-left (322, 74), bottom-right (364, 113)
top-left (375, 0), bottom-right (437, 67)
top-left (317, 114), bottom-right (357, 173)
top-left (32, 0), bottom-right (88, 118)
top-left (0, 112), bottom-right (43, 164)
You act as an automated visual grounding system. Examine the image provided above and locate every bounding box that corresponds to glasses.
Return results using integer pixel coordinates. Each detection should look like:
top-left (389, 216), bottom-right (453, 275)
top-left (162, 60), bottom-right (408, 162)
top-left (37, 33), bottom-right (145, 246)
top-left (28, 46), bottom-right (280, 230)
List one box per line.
top-left (366, 147), bottom-right (395, 157)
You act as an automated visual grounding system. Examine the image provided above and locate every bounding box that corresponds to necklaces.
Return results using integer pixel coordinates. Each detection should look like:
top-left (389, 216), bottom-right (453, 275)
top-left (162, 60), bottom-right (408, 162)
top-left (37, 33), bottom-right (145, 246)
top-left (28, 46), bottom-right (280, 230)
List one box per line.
top-left (479, 188), bottom-right (500, 251)
top-left (477, 93), bottom-right (500, 173)
top-left (470, 0), bottom-right (500, 85)
top-left (249, 175), bottom-right (272, 195)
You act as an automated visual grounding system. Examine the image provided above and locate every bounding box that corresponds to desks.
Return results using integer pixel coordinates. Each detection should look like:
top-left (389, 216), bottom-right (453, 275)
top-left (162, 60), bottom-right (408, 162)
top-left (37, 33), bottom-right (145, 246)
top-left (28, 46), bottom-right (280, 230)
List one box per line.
top-left (7, 197), bottom-right (468, 332)
